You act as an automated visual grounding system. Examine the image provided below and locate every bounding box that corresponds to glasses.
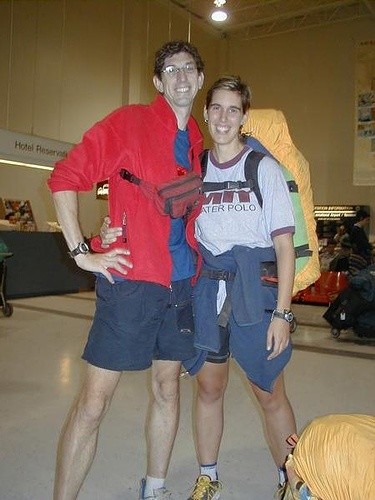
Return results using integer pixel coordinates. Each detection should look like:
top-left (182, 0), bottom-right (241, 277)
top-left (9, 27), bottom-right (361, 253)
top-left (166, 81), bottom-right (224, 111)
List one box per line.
top-left (160, 64), bottom-right (200, 75)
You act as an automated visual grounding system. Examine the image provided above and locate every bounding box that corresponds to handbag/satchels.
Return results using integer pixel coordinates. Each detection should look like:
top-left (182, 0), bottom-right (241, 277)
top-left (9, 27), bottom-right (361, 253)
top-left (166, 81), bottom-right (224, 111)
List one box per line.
top-left (137, 172), bottom-right (204, 219)
top-left (260, 260), bottom-right (278, 288)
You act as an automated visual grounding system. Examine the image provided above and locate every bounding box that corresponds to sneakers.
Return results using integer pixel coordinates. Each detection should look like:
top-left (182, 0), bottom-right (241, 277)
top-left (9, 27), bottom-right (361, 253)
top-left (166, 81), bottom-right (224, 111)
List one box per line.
top-left (187, 475), bottom-right (224, 500)
top-left (275, 483), bottom-right (294, 500)
top-left (139, 478), bottom-right (172, 500)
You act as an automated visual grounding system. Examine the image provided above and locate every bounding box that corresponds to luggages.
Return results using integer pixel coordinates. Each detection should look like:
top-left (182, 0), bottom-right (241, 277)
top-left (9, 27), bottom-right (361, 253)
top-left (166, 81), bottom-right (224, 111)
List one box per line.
top-left (322, 283), bottom-right (372, 338)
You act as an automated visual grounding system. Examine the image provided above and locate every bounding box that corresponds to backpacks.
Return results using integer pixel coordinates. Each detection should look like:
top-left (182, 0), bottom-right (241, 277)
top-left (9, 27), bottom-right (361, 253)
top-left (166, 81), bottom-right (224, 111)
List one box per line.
top-left (197, 108), bottom-right (322, 297)
